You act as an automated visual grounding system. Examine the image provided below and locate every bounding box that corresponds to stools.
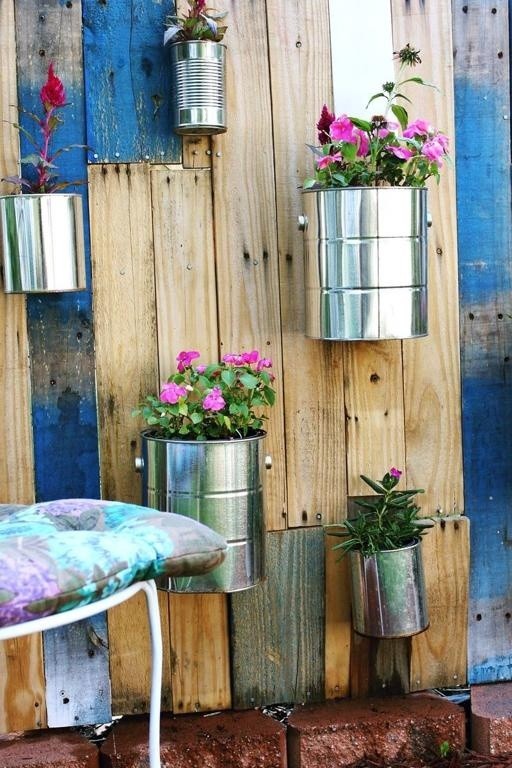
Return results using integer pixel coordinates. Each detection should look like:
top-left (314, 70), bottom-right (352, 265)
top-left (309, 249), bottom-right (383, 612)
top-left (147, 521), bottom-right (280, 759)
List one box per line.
top-left (0, 495), bottom-right (231, 768)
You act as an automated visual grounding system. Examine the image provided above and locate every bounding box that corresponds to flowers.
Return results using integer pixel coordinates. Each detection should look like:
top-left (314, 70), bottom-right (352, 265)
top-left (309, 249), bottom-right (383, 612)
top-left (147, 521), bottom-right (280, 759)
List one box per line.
top-left (162, 0), bottom-right (228, 41)
top-left (137, 347), bottom-right (277, 439)
top-left (325, 467), bottom-right (425, 557)
top-left (2, 61), bottom-right (94, 193)
top-left (303, 43), bottom-right (450, 188)
